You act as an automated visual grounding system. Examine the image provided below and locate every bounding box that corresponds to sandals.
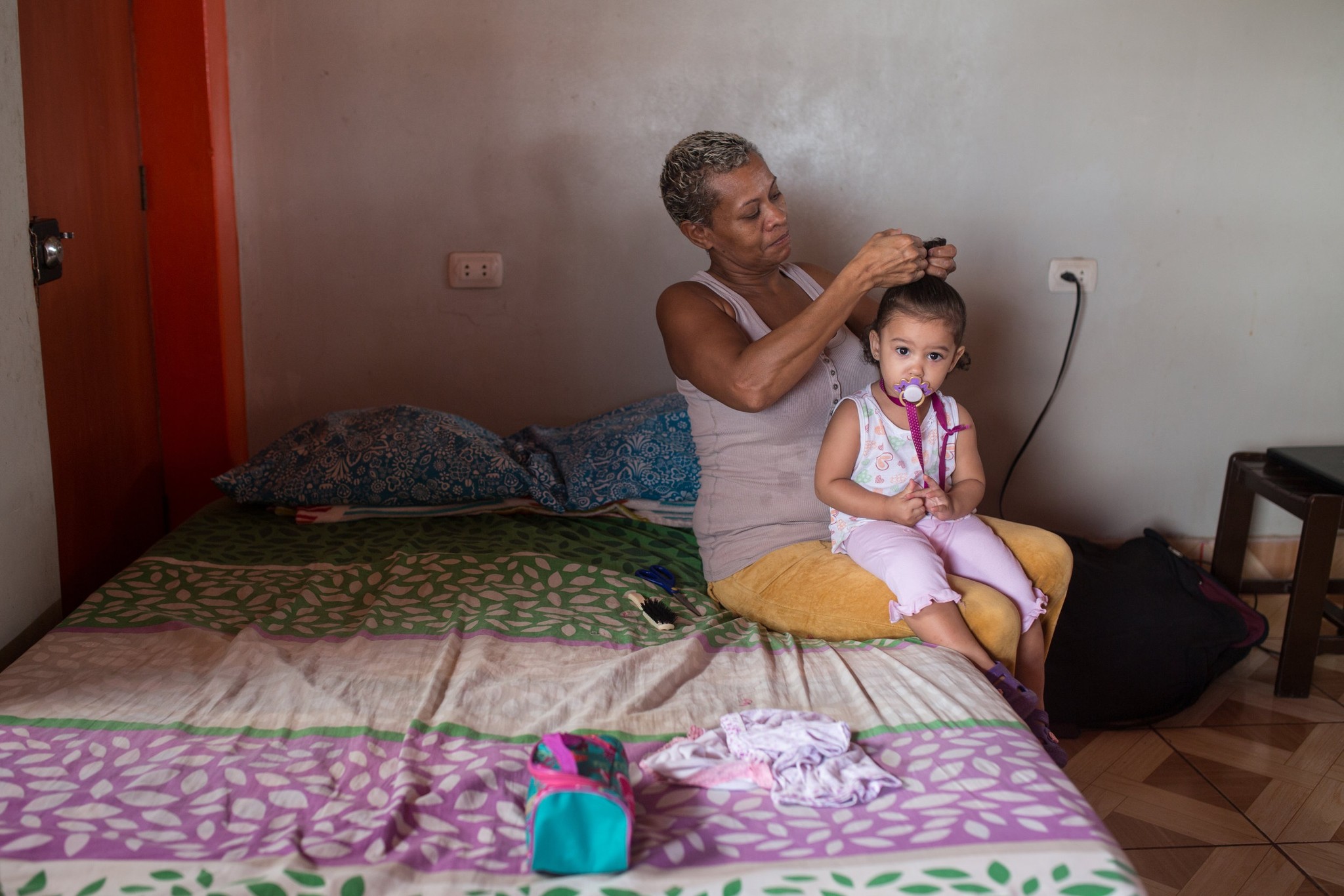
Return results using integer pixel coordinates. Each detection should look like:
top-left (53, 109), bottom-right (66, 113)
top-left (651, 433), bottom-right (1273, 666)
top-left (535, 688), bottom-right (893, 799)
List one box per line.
top-left (1025, 709), bottom-right (1068, 769)
top-left (984, 661), bottom-right (1040, 720)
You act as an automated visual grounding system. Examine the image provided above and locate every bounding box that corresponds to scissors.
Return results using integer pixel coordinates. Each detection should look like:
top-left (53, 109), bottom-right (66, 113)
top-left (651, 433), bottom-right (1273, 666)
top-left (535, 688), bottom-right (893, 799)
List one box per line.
top-left (633, 565), bottom-right (702, 617)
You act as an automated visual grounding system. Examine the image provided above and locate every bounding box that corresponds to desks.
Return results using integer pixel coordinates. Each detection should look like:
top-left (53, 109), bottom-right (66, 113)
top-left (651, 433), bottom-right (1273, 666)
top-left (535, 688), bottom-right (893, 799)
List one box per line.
top-left (1211, 451), bottom-right (1343, 698)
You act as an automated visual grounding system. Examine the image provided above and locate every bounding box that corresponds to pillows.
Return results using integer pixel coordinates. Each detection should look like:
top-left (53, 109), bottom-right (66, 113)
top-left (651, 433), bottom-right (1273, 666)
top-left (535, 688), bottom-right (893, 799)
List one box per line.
top-left (211, 393), bottom-right (703, 513)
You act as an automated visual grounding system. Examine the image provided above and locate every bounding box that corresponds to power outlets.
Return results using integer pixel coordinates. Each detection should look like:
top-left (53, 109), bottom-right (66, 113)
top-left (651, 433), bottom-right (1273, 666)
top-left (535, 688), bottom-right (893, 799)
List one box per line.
top-left (1046, 258), bottom-right (1097, 292)
top-left (448, 252), bottom-right (504, 289)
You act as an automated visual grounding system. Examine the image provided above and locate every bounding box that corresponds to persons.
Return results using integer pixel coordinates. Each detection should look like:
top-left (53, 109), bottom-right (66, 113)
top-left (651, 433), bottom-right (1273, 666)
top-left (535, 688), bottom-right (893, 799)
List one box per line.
top-left (814, 239), bottom-right (1068, 767)
top-left (657, 131), bottom-right (1074, 693)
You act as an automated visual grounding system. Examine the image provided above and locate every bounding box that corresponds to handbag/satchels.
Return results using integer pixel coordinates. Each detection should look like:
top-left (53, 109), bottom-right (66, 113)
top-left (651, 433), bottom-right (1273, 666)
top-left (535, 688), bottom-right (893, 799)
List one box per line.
top-left (526, 732), bottom-right (636, 874)
top-left (1044, 527), bottom-right (1270, 740)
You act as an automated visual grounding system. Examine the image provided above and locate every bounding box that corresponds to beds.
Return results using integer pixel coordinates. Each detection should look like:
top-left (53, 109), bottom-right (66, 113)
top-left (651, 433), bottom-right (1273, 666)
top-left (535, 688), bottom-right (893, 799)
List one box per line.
top-left (0, 501), bottom-right (1150, 895)
